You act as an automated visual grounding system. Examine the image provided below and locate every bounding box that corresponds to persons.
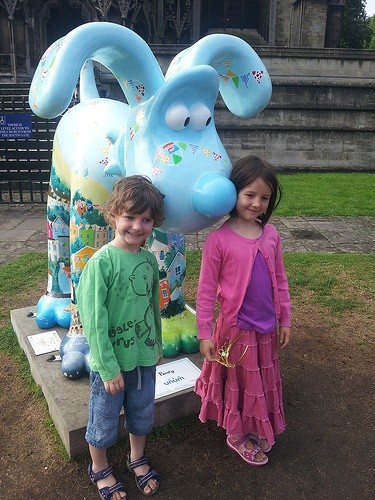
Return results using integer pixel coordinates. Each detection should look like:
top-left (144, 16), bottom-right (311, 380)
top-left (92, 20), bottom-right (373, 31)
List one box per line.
top-left (75, 176), bottom-right (164, 500)
top-left (196, 155), bottom-right (291, 465)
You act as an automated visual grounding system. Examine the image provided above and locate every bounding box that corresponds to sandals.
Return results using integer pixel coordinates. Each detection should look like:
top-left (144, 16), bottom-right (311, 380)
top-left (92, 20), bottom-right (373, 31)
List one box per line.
top-left (127, 451), bottom-right (159, 495)
top-left (251, 434), bottom-right (272, 452)
top-left (226, 436), bottom-right (268, 465)
top-left (88, 463), bottom-right (127, 500)
